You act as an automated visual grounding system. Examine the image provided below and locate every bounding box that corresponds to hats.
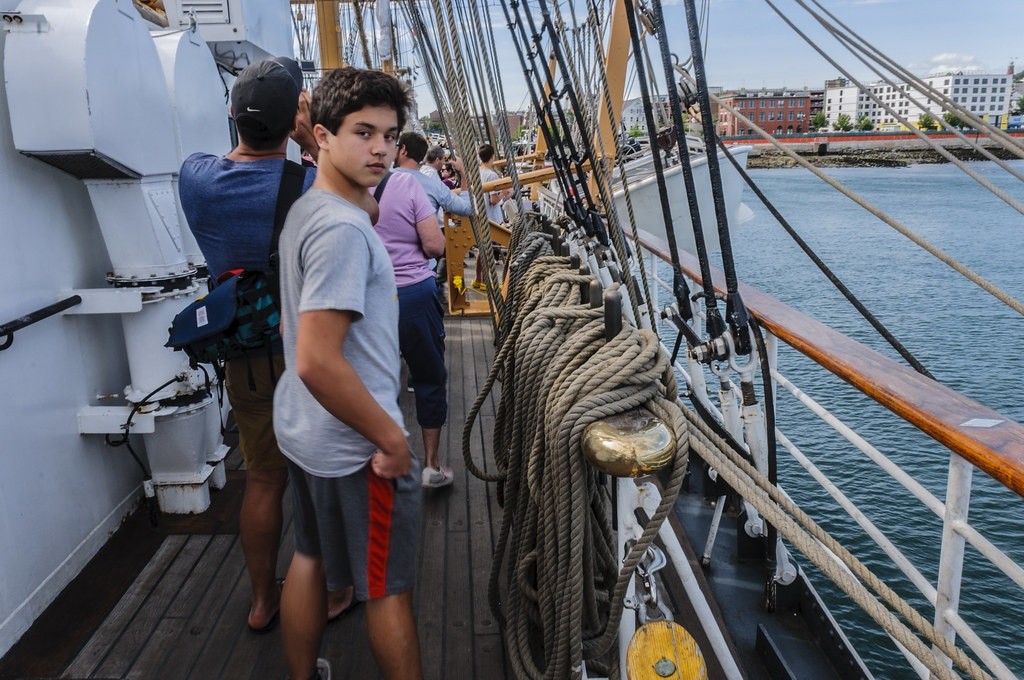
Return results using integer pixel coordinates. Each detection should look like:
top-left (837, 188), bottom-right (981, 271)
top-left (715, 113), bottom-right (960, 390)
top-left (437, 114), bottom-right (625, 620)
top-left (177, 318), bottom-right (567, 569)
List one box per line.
top-left (231, 56), bottom-right (304, 144)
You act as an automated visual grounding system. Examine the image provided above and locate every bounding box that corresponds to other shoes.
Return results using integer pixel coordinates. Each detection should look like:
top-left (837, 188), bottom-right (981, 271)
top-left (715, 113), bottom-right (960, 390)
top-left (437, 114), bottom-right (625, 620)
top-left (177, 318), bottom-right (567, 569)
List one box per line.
top-left (421, 466), bottom-right (454, 488)
top-left (495, 259), bottom-right (500, 265)
top-left (317, 657), bottom-right (330, 680)
top-left (471, 279), bottom-right (503, 290)
top-left (436, 277), bottom-right (447, 285)
top-left (407, 375), bottom-right (415, 392)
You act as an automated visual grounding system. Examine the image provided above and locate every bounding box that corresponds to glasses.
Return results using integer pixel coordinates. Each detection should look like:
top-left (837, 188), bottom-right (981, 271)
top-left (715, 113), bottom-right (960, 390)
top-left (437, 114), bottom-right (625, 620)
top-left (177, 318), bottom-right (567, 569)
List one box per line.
top-left (396, 144), bottom-right (402, 150)
top-left (441, 168), bottom-right (451, 172)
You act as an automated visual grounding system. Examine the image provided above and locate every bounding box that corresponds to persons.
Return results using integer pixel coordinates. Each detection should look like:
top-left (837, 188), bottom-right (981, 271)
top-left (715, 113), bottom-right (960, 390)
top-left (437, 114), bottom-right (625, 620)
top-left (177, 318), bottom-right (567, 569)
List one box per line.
top-left (372, 133), bottom-right (511, 491)
top-left (273, 66), bottom-right (427, 679)
top-left (175, 59), bottom-right (379, 631)
top-left (474, 143), bottom-right (510, 294)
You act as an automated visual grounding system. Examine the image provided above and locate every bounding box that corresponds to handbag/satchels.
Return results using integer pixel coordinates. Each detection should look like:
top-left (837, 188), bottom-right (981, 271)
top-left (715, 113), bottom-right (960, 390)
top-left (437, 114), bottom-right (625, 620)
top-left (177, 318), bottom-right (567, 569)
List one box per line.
top-left (163, 158), bottom-right (307, 364)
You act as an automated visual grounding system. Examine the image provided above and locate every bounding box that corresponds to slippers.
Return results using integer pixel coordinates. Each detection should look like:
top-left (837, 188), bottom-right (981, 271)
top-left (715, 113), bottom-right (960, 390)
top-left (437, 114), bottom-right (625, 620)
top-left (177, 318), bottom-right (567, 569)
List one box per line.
top-left (326, 584), bottom-right (357, 621)
top-left (248, 579), bottom-right (286, 630)
